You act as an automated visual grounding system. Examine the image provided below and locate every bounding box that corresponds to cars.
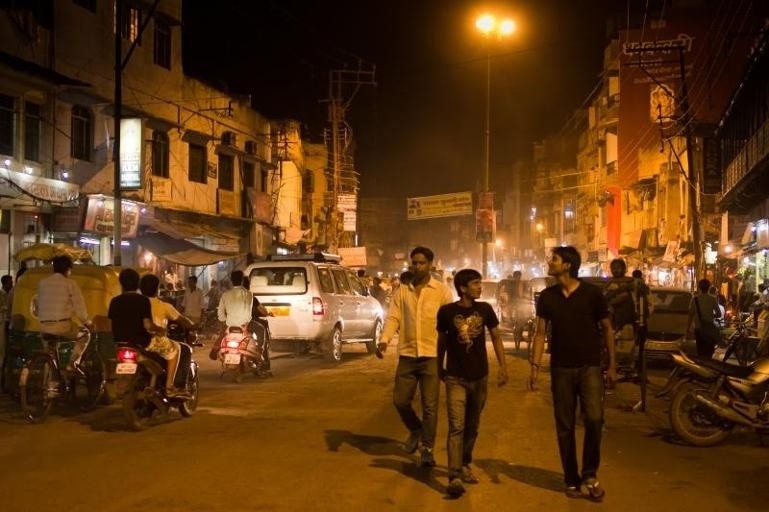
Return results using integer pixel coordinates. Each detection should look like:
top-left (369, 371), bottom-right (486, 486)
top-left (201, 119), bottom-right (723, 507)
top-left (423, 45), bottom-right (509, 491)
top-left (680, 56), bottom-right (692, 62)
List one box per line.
top-left (476, 276), bottom-right (616, 348)
top-left (641, 287), bottom-right (698, 364)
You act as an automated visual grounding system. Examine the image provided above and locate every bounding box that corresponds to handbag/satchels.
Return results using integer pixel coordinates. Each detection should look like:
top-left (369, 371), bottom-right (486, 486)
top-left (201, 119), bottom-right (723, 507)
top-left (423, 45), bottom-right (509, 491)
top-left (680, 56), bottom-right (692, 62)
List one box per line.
top-left (699, 319), bottom-right (722, 344)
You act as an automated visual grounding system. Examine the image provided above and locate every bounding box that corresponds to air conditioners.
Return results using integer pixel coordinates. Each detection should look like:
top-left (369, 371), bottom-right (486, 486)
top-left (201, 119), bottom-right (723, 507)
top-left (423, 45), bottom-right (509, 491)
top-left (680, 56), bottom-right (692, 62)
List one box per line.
top-left (250, 141), bottom-right (259, 155)
top-left (229, 131), bottom-right (240, 147)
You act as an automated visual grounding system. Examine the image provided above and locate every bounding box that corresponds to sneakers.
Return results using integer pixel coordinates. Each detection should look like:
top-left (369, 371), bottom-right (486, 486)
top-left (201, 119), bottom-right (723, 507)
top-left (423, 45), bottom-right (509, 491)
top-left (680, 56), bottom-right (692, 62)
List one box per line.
top-left (583, 476), bottom-right (607, 498)
top-left (67, 367), bottom-right (86, 377)
top-left (420, 448), bottom-right (436, 466)
top-left (461, 463), bottom-right (481, 485)
top-left (565, 480), bottom-right (583, 499)
top-left (166, 386), bottom-right (195, 400)
top-left (401, 422), bottom-right (422, 454)
top-left (446, 478), bottom-right (468, 497)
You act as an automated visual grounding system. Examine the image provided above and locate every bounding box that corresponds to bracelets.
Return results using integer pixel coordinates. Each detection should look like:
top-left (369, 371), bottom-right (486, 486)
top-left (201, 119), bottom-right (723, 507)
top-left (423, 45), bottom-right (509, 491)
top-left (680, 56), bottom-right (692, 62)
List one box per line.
top-left (530, 361), bottom-right (540, 368)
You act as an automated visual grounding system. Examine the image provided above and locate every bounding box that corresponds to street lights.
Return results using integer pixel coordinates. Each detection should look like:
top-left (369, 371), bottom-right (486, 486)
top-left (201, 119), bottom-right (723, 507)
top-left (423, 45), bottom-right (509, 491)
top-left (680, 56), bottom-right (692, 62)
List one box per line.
top-left (473, 12), bottom-right (514, 276)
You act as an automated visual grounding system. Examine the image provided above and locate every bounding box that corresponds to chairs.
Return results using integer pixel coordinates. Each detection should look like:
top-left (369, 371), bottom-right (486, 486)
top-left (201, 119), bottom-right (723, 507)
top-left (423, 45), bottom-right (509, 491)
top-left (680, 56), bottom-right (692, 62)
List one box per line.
top-left (250, 273), bottom-right (306, 291)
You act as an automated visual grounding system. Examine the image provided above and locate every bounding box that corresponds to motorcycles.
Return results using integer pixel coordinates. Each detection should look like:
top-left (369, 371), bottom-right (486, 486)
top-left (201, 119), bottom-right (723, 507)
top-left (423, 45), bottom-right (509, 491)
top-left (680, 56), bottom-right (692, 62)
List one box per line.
top-left (219, 316), bottom-right (276, 381)
top-left (114, 325), bottom-right (204, 430)
top-left (5, 264), bottom-right (121, 398)
top-left (652, 351), bottom-right (769, 446)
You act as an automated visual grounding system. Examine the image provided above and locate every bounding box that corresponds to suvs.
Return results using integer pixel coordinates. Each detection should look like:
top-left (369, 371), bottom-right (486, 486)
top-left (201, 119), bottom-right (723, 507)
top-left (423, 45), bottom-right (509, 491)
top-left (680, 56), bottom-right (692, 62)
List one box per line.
top-left (244, 252), bottom-right (385, 364)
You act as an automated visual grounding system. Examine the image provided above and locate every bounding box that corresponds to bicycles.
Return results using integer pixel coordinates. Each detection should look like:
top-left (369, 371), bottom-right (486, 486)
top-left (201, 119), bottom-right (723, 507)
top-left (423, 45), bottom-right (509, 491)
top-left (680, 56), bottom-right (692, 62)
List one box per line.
top-left (713, 317), bottom-right (755, 366)
top-left (19, 323), bottom-right (105, 425)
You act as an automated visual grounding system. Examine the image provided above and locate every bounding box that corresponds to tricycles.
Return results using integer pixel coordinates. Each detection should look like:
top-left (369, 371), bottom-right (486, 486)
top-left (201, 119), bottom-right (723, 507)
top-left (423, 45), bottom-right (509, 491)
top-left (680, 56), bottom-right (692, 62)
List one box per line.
top-left (602, 317), bottom-right (649, 411)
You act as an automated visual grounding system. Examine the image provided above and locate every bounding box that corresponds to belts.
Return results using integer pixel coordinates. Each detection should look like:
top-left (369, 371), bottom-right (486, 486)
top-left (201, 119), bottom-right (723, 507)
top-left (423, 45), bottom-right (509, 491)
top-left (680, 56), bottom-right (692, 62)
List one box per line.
top-left (39, 318), bottom-right (73, 323)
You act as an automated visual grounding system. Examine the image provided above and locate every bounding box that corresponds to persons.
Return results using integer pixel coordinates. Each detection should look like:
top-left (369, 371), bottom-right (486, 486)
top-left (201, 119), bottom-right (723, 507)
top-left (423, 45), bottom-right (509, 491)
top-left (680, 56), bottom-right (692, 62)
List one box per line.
top-left (357, 263), bottom-right (522, 311)
top-left (602, 255), bottom-right (654, 355)
top-left (140, 274), bottom-right (198, 402)
top-left (39, 253), bottom-right (96, 379)
top-left (108, 268), bottom-right (187, 399)
top-left (435, 269), bottom-right (510, 491)
top-left (687, 278), bottom-right (721, 358)
top-left (5, 269), bottom-right (26, 387)
top-left (0, 274), bottom-right (12, 395)
top-left (159, 276), bottom-right (222, 344)
top-left (710, 269), bottom-right (769, 327)
top-left (242, 275), bottom-right (274, 377)
top-left (526, 245), bottom-right (617, 501)
top-left (376, 245), bottom-right (454, 464)
top-left (208, 270), bottom-right (266, 369)
